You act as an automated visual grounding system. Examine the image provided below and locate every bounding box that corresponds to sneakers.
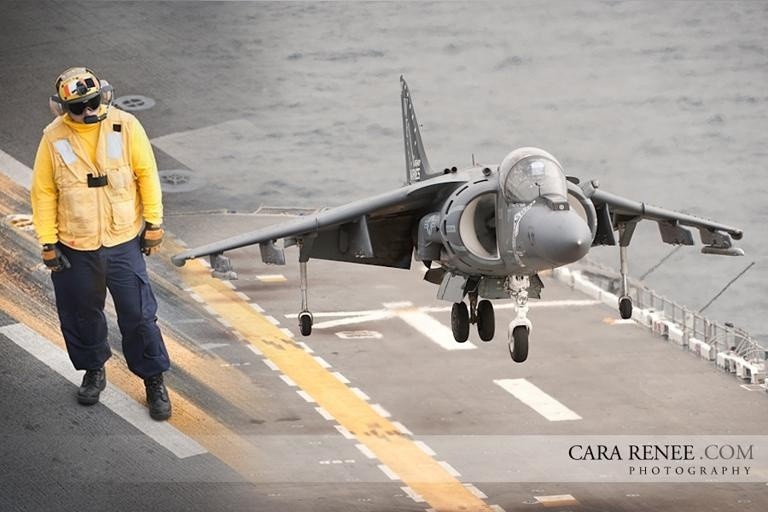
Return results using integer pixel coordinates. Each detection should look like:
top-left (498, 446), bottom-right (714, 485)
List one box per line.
top-left (74, 361), bottom-right (107, 404)
top-left (143, 370), bottom-right (172, 423)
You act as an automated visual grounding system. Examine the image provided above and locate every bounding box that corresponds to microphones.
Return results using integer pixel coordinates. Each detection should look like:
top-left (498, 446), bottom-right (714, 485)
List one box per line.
top-left (83, 102), bottom-right (110, 124)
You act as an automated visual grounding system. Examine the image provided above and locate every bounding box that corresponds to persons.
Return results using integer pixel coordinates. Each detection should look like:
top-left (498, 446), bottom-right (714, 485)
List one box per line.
top-left (31, 66), bottom-right (172, 421)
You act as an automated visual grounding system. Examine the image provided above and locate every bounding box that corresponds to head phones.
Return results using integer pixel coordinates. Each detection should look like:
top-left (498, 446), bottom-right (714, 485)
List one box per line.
top-left (49, 66), bottom-right (113, 116)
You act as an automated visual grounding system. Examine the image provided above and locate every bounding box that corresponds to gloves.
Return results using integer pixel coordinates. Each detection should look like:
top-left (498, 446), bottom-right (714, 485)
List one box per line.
top-left (139, 221), bottom-right (167, 257)
top-left (41, 240), bottom-right (73, 273)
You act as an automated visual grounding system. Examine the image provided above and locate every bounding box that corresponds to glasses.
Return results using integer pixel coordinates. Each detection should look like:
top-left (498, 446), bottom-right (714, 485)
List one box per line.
top-left (65, 95), bottom-right (101, 114)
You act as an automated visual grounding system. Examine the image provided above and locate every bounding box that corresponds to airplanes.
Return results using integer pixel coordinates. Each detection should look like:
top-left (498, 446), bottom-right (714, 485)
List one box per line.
top-left (170, 73), bottom-right (745, 363)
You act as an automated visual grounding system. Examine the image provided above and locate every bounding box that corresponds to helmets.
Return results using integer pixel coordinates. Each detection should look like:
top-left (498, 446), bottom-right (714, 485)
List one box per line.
top-left (55, 65), bottom-right (102, 104)
